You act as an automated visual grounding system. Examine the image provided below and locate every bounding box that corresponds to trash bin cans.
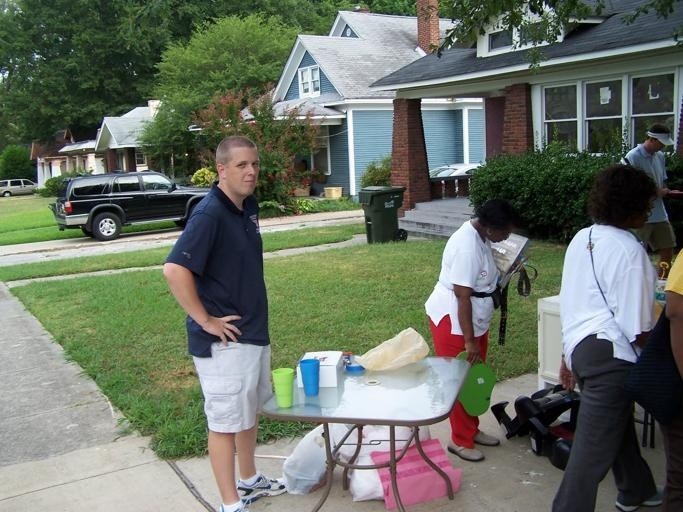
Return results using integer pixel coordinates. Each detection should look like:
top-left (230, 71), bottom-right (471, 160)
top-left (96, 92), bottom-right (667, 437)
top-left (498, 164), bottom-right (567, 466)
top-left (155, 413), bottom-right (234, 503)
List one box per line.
top-left (359, 185), bottom-right (407, 244)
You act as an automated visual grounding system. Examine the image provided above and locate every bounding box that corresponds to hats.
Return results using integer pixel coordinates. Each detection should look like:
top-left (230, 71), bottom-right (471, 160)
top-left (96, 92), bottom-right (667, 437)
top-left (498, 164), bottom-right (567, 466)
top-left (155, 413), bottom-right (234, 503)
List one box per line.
top-left (647, 130), bottom-right (675, 146)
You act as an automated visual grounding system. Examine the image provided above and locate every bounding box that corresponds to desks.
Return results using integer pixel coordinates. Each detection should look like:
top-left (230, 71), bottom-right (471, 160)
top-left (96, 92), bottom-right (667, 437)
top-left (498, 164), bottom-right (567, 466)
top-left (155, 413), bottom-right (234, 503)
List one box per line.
top-left (258, 354), bottom-right (471, 512)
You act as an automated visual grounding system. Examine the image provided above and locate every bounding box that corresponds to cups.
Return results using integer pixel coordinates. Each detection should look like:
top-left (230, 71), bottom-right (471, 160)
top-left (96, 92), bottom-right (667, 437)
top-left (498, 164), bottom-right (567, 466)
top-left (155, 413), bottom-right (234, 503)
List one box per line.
top-left (272, 368), bottom-right (295, 408)
top-left (299, 358), bottom-right (320, 397)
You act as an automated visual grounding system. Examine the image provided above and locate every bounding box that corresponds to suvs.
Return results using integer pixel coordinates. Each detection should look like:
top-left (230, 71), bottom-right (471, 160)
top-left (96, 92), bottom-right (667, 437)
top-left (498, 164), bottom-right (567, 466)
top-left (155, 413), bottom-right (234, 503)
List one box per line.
top-left (47, 170), bottom-right (213, 241)
top-left (0, 178), bottom-right (38, 198)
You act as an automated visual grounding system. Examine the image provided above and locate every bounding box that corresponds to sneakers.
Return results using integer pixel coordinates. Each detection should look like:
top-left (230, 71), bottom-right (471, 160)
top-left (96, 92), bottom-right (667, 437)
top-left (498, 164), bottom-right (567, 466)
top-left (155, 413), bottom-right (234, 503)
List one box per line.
top-left (615, 484), bottom-right (665, 511)
top-left (219, 502), bottom-right (251, 512)
top-left (236, 473), bottom-right (287, 505)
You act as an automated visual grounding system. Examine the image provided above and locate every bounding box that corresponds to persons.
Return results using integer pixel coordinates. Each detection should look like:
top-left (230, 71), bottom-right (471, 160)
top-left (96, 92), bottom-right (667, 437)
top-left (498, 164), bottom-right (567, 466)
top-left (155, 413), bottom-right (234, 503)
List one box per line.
top-left (664, 248), bottom-right (683, 512)
top-left (424, 199), bottom-right (524, 462)
top-left (551, 164), bottom-right (665, 512)
top-left (163, 135), bottom-right (288, 512)
top-left (618, 124), bottom-right (683, 281)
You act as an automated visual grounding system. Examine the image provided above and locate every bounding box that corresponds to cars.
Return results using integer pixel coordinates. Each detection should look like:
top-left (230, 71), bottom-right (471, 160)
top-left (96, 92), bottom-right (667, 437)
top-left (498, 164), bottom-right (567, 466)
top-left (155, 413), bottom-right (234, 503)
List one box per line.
top-left (428, 163), bottom-right (486, 175)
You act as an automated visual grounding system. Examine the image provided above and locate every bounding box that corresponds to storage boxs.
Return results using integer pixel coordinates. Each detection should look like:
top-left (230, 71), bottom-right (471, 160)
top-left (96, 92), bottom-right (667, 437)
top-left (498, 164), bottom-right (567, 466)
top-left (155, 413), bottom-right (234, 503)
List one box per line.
top-left (324, 187), bottom-right (343, 198)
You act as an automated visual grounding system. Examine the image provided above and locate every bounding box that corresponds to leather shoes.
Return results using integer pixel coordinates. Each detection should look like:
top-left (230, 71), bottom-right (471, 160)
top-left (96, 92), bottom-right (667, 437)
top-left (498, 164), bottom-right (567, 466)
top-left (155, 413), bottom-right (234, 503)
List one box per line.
top-left (447, 436), bottom-right (485, 461)
top-left (473, 431), bottom-right (500, 447)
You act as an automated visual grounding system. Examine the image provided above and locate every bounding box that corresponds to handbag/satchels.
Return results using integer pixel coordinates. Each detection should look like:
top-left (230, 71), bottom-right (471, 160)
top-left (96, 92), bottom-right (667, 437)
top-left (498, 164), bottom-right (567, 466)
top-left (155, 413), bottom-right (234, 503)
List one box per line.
top-left (621, 304), bottom-right (683, 431)
top-left (371, 439), bottom-right (462, 510)
